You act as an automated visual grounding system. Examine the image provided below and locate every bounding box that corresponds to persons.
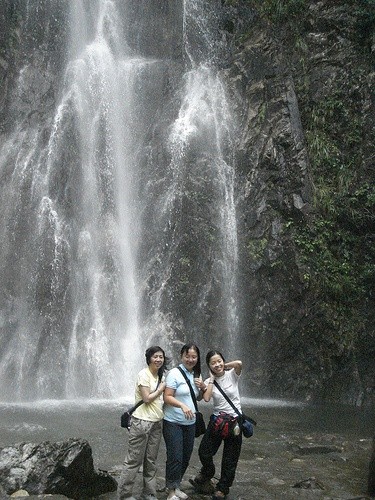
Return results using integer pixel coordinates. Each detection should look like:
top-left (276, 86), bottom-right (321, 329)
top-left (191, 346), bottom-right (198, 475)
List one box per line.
top-left (196, 351), bottom-right (243, 500)
top-left (162, 343), bottom-right (207, 500)
top-left (119, 346), bottom-right (166, 500)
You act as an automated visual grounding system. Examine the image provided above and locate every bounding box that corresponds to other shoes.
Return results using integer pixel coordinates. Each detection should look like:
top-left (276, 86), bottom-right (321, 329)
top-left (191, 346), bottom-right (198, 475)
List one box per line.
top-left (119, 495), bottom-right (137, 500)
top-left (173, 488), bottom-right (188, 500)
top-left (194, 471), bottom-right (209, 483)
top-left (140, 493), bottom-right (159, 500)
top-left (213, 489), bottom-right (226, 500)
top-left (167, 495), bottom-right (181, 500)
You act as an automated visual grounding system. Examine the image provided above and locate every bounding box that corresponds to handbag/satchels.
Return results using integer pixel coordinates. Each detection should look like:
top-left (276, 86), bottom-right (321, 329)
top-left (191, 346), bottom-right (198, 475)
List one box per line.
top-left (195, 411), bottom-right (206, 437)
top-left (237, 411), bottom-right (257, 438)
top-left (120, 411), bottom-right (131, 428)
